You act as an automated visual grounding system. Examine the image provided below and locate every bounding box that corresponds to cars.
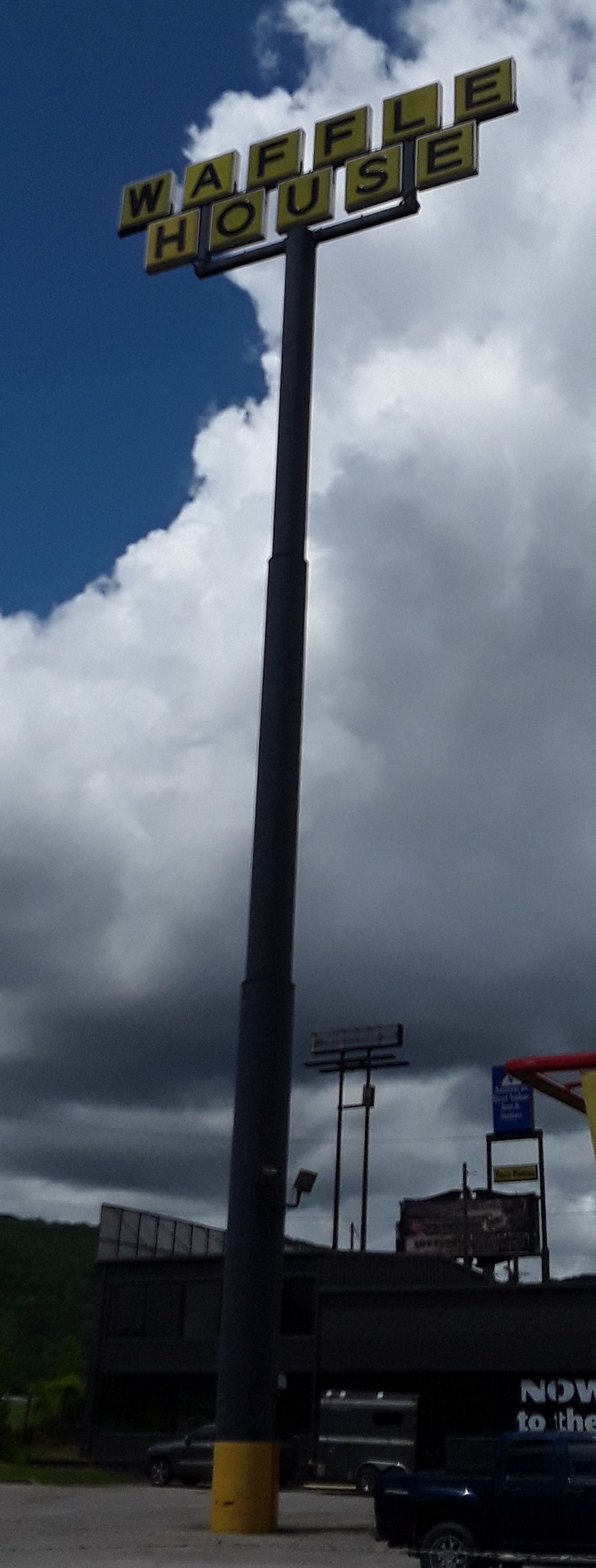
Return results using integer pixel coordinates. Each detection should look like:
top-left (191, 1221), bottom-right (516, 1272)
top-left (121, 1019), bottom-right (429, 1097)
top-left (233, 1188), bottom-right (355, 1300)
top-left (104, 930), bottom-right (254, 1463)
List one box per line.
top-left (144, 1423), bottom-right (298, 1489)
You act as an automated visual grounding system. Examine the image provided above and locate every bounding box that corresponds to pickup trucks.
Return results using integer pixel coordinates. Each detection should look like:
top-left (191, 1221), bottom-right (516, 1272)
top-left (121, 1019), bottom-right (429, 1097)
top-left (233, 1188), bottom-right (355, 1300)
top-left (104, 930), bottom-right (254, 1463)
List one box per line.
top-left (374, 1430), bottom-right (596, 1567)
